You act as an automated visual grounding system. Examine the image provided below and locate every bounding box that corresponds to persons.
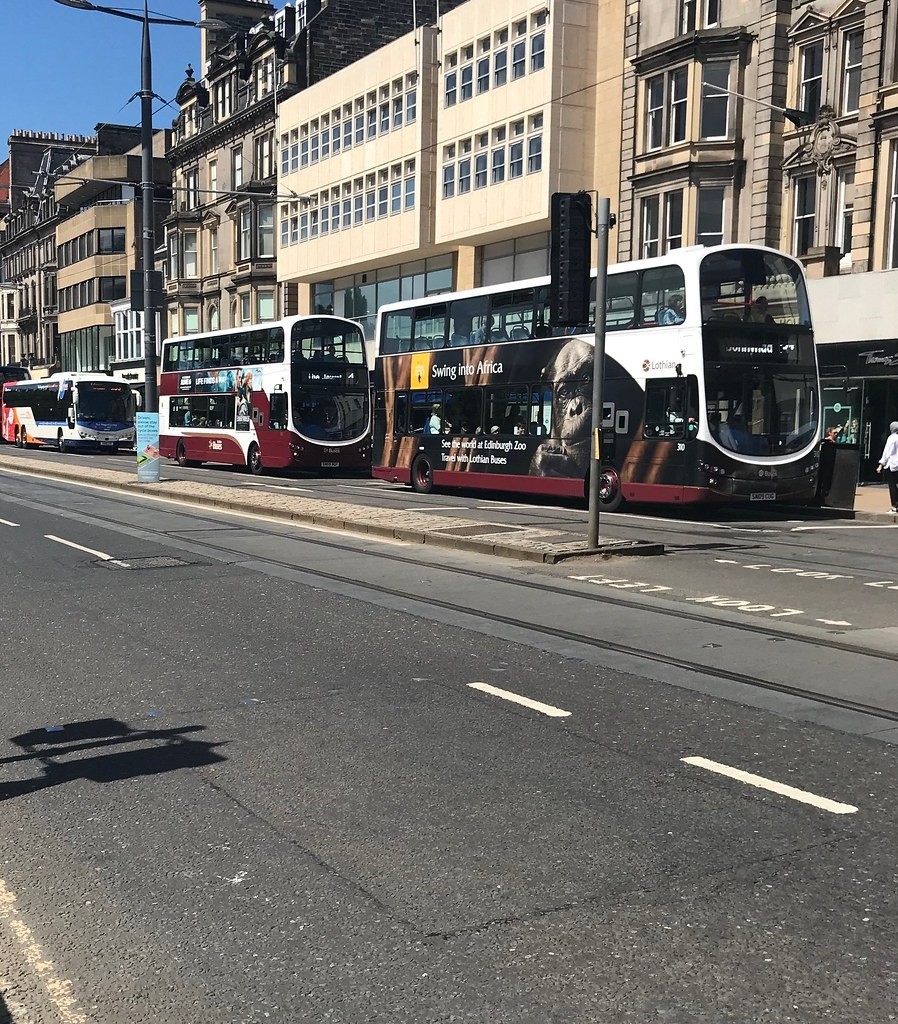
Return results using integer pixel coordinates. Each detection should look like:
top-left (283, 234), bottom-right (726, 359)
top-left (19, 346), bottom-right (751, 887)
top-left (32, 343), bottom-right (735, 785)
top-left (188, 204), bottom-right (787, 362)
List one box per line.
top-left (324, 346), bottom-right (338, 361)
top-left (667, 390), bottom-right (696, 434)
top-left (397, 402), bottom-right (547, 436)
top-left (77, 401), bottom-right (94, 419)
top-left (183, 405), bottom-right (206, 426)
top-left (820, 427), bottom-right (838, 451)
top-left (663, 295), bottom-right (684, 325)
top-left (748, 296), bottom-right (775, 322)
top-left (877, 421), bottom-right (898, 514)
top-left (475, 316), bottom-right (496, 344)
top-left (208, 368), bottom-right (253, 415)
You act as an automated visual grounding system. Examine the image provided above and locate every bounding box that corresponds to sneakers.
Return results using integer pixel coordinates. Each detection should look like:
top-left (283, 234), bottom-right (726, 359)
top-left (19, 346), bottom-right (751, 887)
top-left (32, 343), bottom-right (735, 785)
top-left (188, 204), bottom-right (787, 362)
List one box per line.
top-left (886, 508), bottom-right (898, 513)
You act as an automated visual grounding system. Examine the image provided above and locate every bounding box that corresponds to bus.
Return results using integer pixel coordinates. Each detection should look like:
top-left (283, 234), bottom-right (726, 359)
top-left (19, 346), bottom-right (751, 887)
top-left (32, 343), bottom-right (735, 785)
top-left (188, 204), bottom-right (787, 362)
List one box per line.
top-left (0, 365), bottom-right (32, 403)
top-left (1, 371), bottom-right (143, 452)
top-left (159, 313), bottom-right (381, 476)
top-left (373, 243), bottom-right (848, 513)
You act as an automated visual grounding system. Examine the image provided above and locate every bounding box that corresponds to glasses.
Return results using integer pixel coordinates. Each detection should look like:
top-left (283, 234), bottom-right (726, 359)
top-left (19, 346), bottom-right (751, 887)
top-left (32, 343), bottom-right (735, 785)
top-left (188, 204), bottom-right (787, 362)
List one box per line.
top-left (831, 432), bottom-right (837, 434)
